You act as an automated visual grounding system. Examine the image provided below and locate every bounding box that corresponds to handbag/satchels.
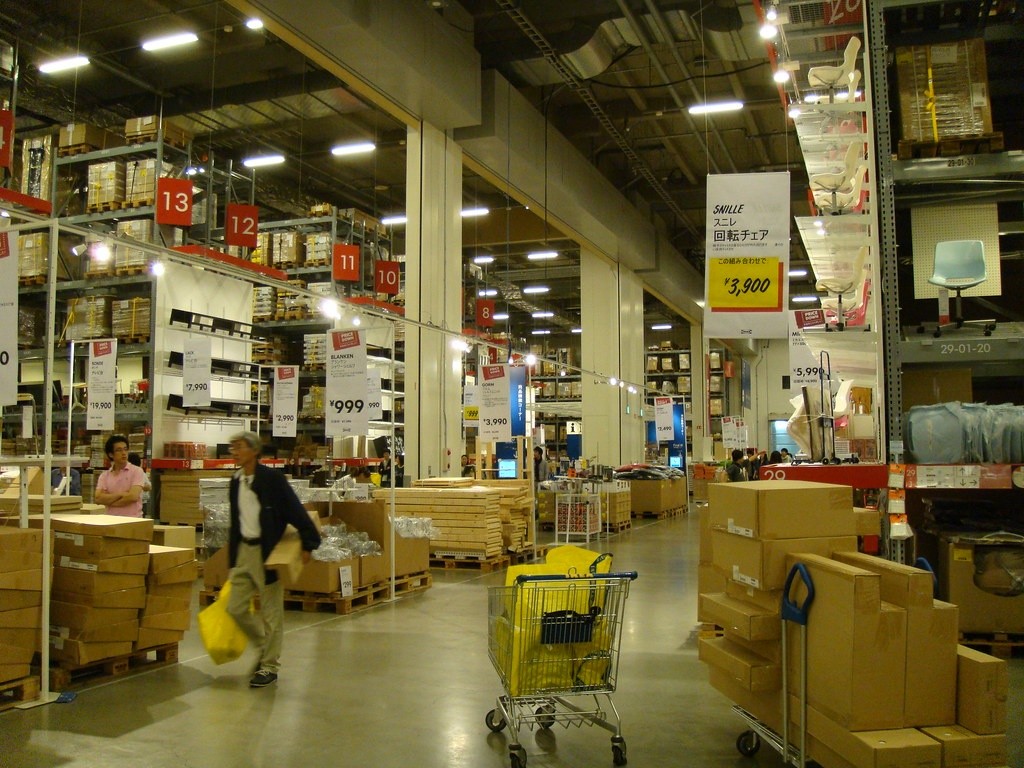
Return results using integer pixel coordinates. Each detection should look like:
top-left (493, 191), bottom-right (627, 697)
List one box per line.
top-left (196, 578), bottom-right (255, 666)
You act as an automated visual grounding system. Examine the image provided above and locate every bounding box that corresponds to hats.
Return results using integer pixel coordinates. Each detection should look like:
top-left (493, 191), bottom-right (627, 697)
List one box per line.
top-left (732, 450), bottom-right (743, 461)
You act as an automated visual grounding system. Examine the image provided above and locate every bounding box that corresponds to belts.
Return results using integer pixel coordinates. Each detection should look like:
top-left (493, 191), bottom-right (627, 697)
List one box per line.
top-left (241, 536), bottom-right (263, 546)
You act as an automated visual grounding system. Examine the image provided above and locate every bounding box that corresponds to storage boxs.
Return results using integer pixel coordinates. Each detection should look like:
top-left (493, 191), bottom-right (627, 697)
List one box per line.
top-left (571, 381), bottom-right (582, 398)
top-left (702, 527), bottom-right (860, 599)
top-left (696, 594), bottom-right (780, 642)
top-left (601, 477), bottom-right (688, 534)
top-left (709, 374), bottom-right (724, 393)
top-left (693, 636), bottom-right (782, 693)
top-left (529, 344), bottom-right (543, 354)
top-left (720, 577), bottom-right (785, 611)
top-left (0, 107), bottom-right (433, 709)
top-left (647, 380), bottom-right (657, 393)
top-left (721, 628), bottom-right (784, 661)
top-left (834, 549), bottom-right (956, 728)
top-left (678, 401), bottom-right (692, 413)
top-left (896, 37), bottom-right (994, 141)
top-left (558, 382), bottom-right (572, 397)
top-left (543, 360), bottom-right (557, 375)
top-left (662, 357), bottom-right (675, 371)
top-left (778, 689), bottom-right (943, 768)
top-left (711, 352), bottom-right (722, 370)
top-left (660, 381), bottom-right (676, 392)
top-left (701, 480), bottom-right (861, 541)
top-left (660, 339), bottom-right (675, 350)
top-left (705, 666), bottom-right (853, 768)
top-left (544, 425), bottom-right (555, 439)
top-left (785, 552), bottom-right (908, 732)
top-left (557, 348), bottom-right (576, 375)
top-left (543, 381), bottom-right (556, 398)
top-left (958, 642), bottom-right (1012, 734)
top-left (709, 397), bottom-right (724, 416)
top-left (676, 376), bottom-right (691, 394)
top-left (646, 355), bottom-right (659, 372)
top-left (677, 354), bottom-right (690, 370)
top-left (920, 726), bottom-right (1009, 766)
top-left (533, 381), bottom-right (542, 398)
top-left (559, 426), bottom-right (568, 439)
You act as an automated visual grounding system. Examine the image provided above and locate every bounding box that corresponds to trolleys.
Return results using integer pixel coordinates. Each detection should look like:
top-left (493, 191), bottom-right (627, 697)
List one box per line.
top-left (730, 561), bottom-right (815, 768)
top-left (487, 569), bottom-right (638, 768)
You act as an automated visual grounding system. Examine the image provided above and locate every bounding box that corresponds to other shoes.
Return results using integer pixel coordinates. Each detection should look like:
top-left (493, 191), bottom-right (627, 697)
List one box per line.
top-left (250, 670), bottom-right (278, 689)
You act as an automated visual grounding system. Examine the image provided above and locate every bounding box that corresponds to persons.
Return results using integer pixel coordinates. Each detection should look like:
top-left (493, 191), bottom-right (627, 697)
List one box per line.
top-left (395, 455), bottom-right (404, 487)
top-left (482, 454), bottom-right (497, 479)
top-left (331, 466), bottom-right (372, 489)
top-left (728, 448), bottom-right (794, 482)
top-left (534, 446), bottom-right (548, 521)
top-left (221, 431), bottom-right (322, 688)
top-left (95, 435), bottom-right (152, 519)
top-left (2, 423), bottom-right (16, 439)
top-left (378, 449), bottom-right (391, 488)
top-left (461, 455), bottom-right (472, 477)
top-left (51, 467), bottom-right (81, 496)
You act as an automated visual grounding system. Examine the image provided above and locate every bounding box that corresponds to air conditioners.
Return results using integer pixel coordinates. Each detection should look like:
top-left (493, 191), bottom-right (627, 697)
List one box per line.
top-left (530, 326), bottom-right (553, 337)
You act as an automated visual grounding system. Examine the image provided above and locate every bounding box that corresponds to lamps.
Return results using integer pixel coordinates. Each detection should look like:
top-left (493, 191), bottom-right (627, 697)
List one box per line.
top-left (480, 288), bottom-right (500, 296)
top-left (520, 282), bottom-right (556, 298)
top-left (242, 152), bottom-right (290, 169)
top-left (463, 205), bottom-right (495, 220)
top-left (651, 321), bottom-right (675, 331)
top-left (379, 213), bottom-right (406, 226)
top-left (759, 21), bottom-right (781, 40)
top-left (137, 31), bottom-right (201, 56)
top-left (533, 311), bottom-right (552, 318)
top-left (527, 250), bottom-right (559, 262)
top-left (329, 140), bottom-right (379, 158)
top-left (32, 53), bottom-right (92, 79)
top-left (492, 312), bottom-right (511, 322)
top-left (474, 256), bottom-right (494, 265)
top-left (604, 375), bottom-right (640, 395)
top-left (773, 64), bottom-right (791, 83)
top-left (688, 100), bottom-right (743, 117)
top-left (765, 1), bottom-right (778, 21)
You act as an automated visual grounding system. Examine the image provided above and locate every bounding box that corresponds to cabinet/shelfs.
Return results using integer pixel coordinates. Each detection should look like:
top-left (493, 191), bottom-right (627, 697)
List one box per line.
top-left (0, 93), bottom-right (273, 525)
top-left (255, 203), bottom-right (406, 433)
top-left (645, 345), bottom-right (730, 442)
top-left (529, 375), bottom-right (582, 462)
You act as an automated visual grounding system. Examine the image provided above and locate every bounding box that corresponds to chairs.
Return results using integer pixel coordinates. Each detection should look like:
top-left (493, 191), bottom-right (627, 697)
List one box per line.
top-left (785, 35), bottom-right (868, 467)
top-left (916, 238), bottom-right (998, 338)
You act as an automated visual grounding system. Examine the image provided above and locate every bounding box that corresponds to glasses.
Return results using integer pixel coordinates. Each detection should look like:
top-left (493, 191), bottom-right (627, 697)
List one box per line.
top-left (112, 447), bottom-right (128, 452)
top-left (228, 447), bottom-right (250, 453)
